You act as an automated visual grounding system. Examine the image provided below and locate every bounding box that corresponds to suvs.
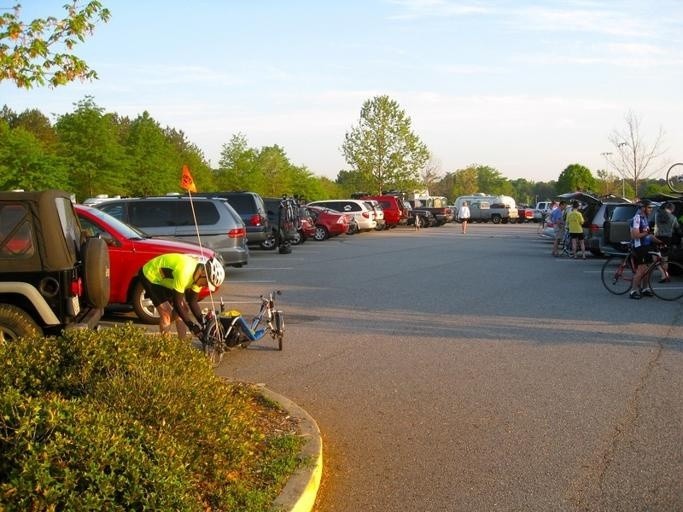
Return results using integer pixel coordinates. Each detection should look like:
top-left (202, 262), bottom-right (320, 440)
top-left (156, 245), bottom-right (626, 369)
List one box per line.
top-left (0, 191), bottom-right (111, 347)
top-left (537, 193), bottom-right (682, 276)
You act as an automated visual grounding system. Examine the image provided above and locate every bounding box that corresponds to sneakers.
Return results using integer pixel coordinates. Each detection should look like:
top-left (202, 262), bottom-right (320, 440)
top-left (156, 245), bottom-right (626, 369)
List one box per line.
top-left (629, 288), bottom-right (654, 300)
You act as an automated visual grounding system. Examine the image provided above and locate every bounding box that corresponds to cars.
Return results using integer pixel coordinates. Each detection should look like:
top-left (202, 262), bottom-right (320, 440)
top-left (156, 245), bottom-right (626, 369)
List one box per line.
top-left (67, 200), bottom-right (225, 326)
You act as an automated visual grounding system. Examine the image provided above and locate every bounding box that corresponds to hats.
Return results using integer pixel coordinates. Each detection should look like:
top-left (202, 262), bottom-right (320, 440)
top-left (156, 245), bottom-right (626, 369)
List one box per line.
top-left (637, 199), bottom-right (657, 209)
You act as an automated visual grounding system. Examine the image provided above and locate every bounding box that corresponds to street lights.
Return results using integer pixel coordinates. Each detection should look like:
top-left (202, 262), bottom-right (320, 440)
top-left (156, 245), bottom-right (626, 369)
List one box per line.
top-left (617, 142), bottom-right (627, 198)
top-left (601, 152), bottom-right (613, 194)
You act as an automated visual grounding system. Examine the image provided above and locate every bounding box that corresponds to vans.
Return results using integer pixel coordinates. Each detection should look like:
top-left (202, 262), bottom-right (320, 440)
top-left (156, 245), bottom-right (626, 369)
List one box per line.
top-left (88, 195), bottom-right (249, 268)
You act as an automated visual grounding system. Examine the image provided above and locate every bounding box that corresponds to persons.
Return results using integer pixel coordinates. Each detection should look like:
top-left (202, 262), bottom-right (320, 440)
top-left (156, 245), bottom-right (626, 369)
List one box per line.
top-left (551, 201), bottom-right (566, 257)
top-left (140, 252), bottom-right (225, 352)
top-left (549, 200), bottom-right (558, 211)
top-left (415, 215), bottom-right (421, 231)
top-left (652, 203), bottom-right (679, 284)
top-left (629, 199), bottom-right (663, 300)
top-left (458, 201), bottom-right (470, 233)
top-left (545, 204), bottom-right (554, 227)
top-left (566, 201), bottom-right (586, 260)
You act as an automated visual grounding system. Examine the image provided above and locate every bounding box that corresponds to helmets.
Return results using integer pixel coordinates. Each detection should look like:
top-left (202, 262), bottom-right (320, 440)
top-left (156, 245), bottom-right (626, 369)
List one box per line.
top-left (206, 257), bottom-right (226, 292)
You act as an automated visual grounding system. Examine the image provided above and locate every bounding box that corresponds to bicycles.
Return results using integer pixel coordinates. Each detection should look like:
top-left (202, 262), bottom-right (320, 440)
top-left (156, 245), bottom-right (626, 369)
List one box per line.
top-left (666, 162), bottom-right (683, 194)
top-left (201, 287), bottom-right (287, 368)
top-left (602, 240), bottom-right (682, 301)
